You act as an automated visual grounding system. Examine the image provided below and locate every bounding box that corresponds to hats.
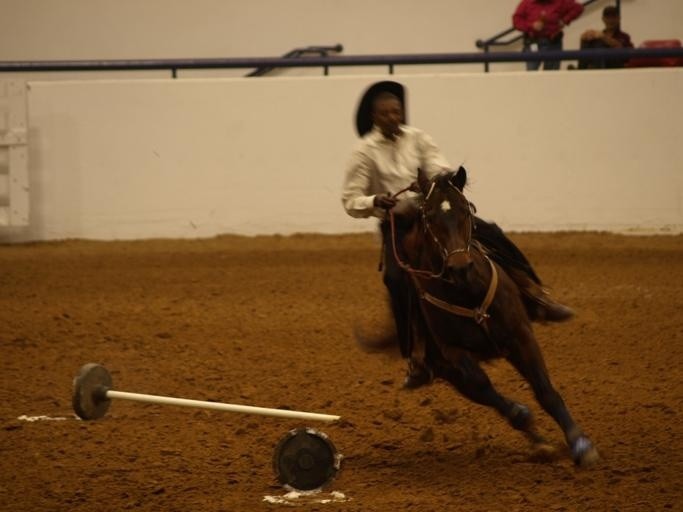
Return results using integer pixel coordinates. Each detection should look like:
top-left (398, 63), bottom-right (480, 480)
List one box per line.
top-left (355, 81), bottom-right (406, 137)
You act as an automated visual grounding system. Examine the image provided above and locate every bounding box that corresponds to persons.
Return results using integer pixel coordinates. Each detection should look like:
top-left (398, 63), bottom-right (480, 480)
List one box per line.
top-left (340, 80), bottom-right (457, 394)
top-left (579, 7), bottom-right (636, 70)
top-left (511, 1), bottom-right (582, 71)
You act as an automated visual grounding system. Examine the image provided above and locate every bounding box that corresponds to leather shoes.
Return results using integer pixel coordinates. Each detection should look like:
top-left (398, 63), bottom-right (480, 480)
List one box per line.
top-left (405, 361), bottom-right (438, 390)
top-left (528, 301), bottom-right (572, 321)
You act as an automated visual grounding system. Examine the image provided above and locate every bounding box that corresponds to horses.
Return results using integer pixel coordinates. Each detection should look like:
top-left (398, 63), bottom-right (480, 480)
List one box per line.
top-left (371, 164), bottom-right (594, 467)
